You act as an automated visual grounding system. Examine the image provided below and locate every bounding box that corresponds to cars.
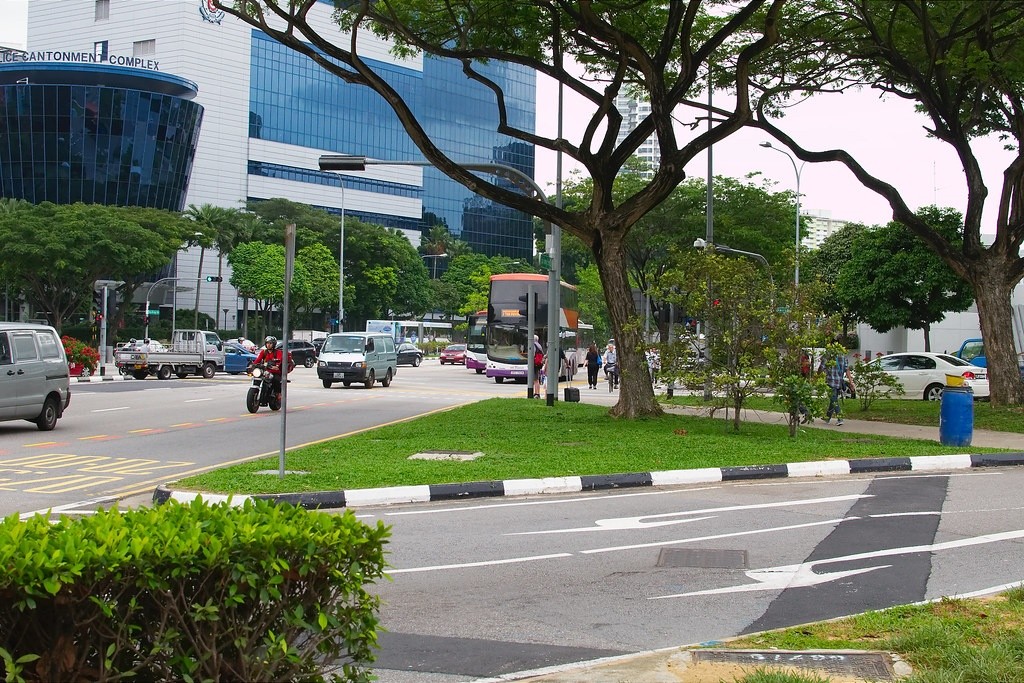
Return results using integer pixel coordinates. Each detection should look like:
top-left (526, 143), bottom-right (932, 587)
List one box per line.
top-left (222, 341), bottom-right (261, 372)
top-left (0, 321), bottom-right (71, 432)
top-left (393, 344), bottom-right (425, 367)
top-left (440, 343), bottom-right (466, 365)
top-left (261, 339), bottom-right (316, 369)
top-left (841, 352), bottom-right (991, 401)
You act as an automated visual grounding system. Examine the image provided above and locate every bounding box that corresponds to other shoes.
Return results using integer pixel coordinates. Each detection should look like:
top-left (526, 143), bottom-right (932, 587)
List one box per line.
top-left (276, 393), bottom-right (281, 401)
top-left (534, 394), bottom-right (540, 399)
top-left (589, 386), bottom-right (596, 389)
top-left (614, 385), bottom-right (618, 389)
top-left (604, 376), bottom-right (609, 380)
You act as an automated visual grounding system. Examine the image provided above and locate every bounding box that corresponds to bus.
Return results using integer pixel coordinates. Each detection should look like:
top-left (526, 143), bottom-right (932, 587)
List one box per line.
top-left (465, 309), bottom-right (488, 374)
top-left (483, 269), bottom-right (583, 381)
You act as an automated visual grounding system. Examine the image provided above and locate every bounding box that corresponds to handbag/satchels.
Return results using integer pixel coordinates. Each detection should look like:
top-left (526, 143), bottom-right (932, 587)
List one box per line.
top-left (597, 351), bottom-right (602, 365)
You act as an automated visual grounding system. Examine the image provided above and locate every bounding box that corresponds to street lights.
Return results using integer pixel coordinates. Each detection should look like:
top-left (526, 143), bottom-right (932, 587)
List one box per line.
top-left (759, 140), bottom-right (810, 376)
top-left (693, 236), bottom-right (776, 376)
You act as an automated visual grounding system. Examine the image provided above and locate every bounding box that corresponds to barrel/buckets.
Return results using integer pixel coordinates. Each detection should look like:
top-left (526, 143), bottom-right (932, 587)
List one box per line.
top-left (938, 386), bottom-right (974, 447)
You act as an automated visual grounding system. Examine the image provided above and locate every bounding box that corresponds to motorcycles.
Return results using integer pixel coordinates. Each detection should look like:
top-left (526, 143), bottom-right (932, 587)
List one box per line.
top-left (244, 354), bottom-right (289, 413)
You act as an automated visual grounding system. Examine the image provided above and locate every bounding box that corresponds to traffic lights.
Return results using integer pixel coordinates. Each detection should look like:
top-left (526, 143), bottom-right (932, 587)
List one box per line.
top-left (207, 276), bottom-right (222, 282)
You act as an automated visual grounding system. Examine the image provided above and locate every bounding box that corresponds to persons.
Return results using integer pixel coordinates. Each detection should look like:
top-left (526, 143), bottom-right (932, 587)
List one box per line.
top-left (127, 339), bottom-right (153, 351)
top-left (815, 337), bottom-right (856, 425)
top-left (251, 336), bottom-right (283, 400)
top-left (533, 334), bottom-right (659, 400)
top-left (236, 338), bottom-right (255, 349)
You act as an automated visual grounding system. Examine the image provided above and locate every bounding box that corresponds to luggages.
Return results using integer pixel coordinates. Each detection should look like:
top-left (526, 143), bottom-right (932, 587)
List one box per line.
top-left (565, 366), bottom-right (580, 402)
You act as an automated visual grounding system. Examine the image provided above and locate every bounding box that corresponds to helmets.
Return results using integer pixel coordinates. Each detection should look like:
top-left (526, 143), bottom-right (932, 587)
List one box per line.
top-left (144, 338), bottom-right (150, 343)
top-left (129, 338), bottom-right (136, 343)
top-left (238, 337), bottom-right (245, 343)
top-left (265, 336), bottom-right (277, 348)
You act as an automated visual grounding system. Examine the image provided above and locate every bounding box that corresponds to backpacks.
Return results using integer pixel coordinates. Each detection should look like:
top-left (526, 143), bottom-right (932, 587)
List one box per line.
top-left (534, 342), bottom-right (544, 367)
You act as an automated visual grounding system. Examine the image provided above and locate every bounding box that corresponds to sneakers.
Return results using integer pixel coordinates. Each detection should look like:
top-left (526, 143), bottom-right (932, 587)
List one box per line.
top-left (836, 421), bottom-right (843, 426)
top-left (821, 417), bottom-right (830, 424)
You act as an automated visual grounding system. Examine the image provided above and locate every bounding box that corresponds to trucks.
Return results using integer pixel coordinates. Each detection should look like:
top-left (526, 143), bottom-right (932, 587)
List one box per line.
top-left (113, 328), bottom-right (226, 379)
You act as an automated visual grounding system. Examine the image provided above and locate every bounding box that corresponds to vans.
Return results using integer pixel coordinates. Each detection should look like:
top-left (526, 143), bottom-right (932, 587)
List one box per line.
top-left (317, 330), bottom-right (397, 389)
